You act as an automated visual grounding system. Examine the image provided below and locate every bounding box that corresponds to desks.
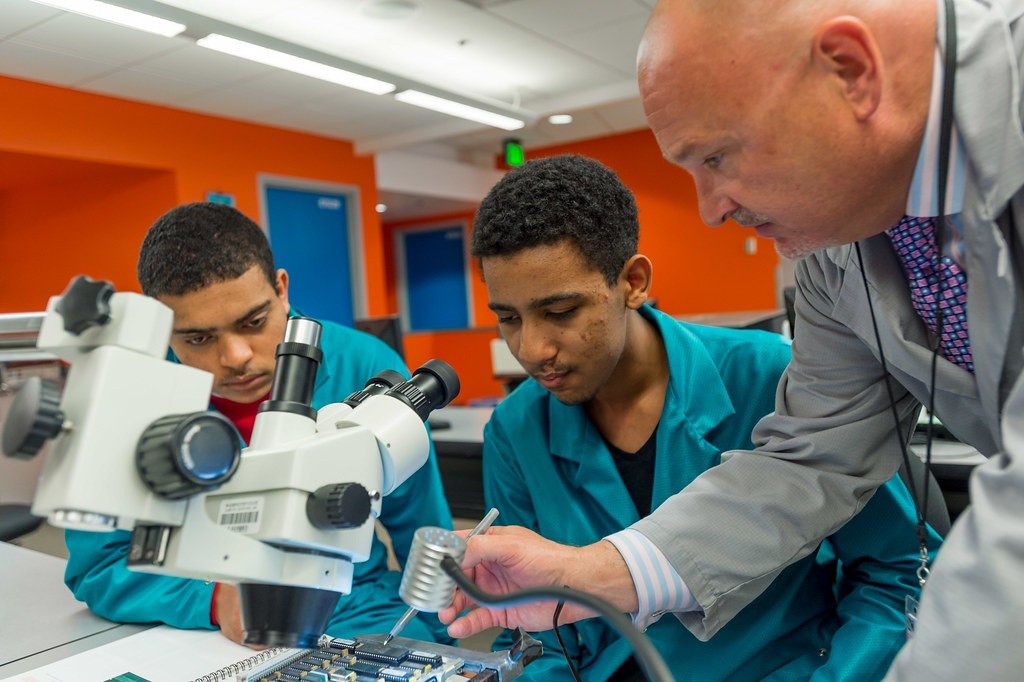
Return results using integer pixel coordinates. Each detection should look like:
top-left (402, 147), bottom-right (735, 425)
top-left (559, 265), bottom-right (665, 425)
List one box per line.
top-left (2, 338), bottom-right (988, 559)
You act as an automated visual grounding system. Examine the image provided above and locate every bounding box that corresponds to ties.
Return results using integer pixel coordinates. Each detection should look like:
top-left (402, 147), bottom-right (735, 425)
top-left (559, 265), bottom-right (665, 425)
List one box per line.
top-left (884, 215), bottom-right (975, 375)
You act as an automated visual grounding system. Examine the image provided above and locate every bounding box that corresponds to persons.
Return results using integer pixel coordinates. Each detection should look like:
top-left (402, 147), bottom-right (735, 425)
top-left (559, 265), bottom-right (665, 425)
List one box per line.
top-left (437, 1), bottom-right (1024, 682)
top-left (64, 201), bottom-right (455, 645)
top-left (473, 156), bottom-right (943, 682)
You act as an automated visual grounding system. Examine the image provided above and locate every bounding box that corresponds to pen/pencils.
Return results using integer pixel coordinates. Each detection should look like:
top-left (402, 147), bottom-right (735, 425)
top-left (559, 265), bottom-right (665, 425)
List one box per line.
top-left (383, 506), bottom-right (501, 646)
